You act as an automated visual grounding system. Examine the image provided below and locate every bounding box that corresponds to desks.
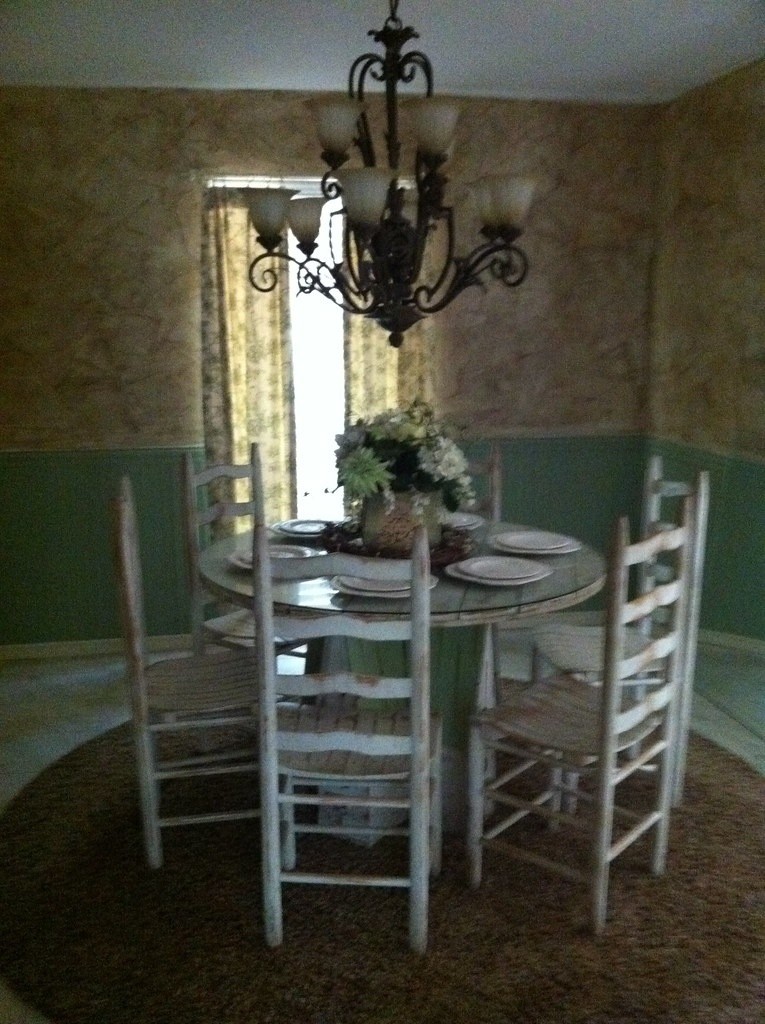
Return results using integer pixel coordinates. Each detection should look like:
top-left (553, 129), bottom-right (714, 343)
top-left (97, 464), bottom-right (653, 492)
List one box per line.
top-left (196, 511), bottom-right (606, 845)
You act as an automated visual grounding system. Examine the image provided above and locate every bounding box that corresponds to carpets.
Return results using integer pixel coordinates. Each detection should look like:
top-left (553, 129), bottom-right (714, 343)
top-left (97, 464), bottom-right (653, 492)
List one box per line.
top-left (0, 719), bottom-right (765, 1024)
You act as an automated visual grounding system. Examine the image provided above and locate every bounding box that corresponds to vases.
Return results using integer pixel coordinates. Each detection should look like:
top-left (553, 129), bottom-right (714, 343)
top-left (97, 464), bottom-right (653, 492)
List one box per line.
top-left (361, 486), bottom-right (443, 551)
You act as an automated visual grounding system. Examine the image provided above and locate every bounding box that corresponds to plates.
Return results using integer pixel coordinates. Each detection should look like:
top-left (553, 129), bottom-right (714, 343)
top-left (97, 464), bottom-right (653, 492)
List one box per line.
top-left (228, 551), bottom-right (253, 569)
top-left (458, 556), bottom-right (542, 579)
top-left (491, 531), bottom-right (582, 553)
top-left (445, 562), bottom-right (552, 586)
top-left (280, 519), bottom-right (326, 533)
top-left (271, 523), bottom-right (323, 537)
top-left (496, 533), bottom-right (572, 550)
top-left (456, 513), bottom-right (485, 529)
top-left (451, 512), bottom-right (478, 527)
top-left (331, 574), bottom-right (439, 598)
top-left (339, 576), bottom-right (411, 591)
top-left (240, 545), bottom-right (328, 562)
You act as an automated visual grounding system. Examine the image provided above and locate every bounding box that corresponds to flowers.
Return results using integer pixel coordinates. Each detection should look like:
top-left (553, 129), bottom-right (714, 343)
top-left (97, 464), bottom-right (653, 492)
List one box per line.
top-left (336, 397), bottom-right (476, 518)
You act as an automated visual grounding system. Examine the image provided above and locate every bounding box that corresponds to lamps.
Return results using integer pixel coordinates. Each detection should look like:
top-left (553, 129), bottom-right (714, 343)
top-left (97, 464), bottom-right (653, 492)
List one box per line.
top-left (238, 0), bottom-right (536, 349)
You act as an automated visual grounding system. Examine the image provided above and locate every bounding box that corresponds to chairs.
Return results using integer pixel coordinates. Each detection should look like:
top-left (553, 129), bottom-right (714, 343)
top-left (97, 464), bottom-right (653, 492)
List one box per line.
top-left (113, 476), bottom-right (265, 871)
top-left (251, 524), bottom-right (443, 955)
top-left (527, 454), bottom-right (711, 810)
top-left (181, 443), bottom-right (326, 707)
top-left (467, 495), bottom-right (697, 937)
